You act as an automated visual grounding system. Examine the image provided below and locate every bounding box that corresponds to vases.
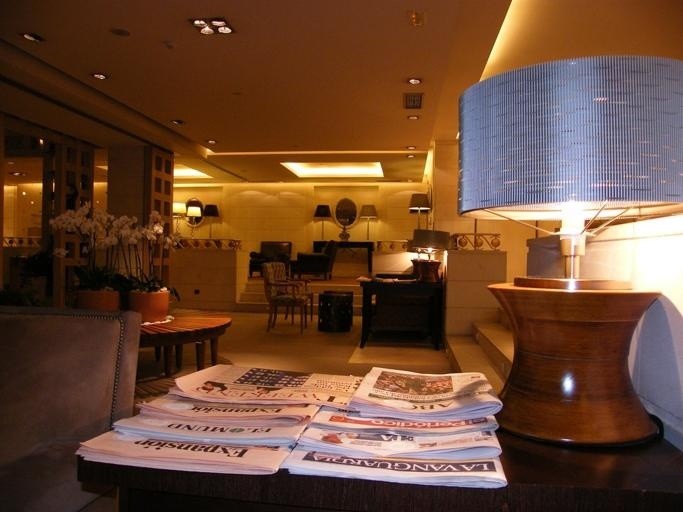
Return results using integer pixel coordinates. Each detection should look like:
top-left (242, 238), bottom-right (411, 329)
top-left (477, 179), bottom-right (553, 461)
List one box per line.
top-left (129, 290), bottom-right (170, 323)
top-left (79, 290), bottom-right (120, 310)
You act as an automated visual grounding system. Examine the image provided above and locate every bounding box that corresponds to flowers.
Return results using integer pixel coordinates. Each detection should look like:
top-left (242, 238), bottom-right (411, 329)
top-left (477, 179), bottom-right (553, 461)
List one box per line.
top-left (126, 209), bottom-right (168, 292)
top-left (49, 199), bottom-right (126, 290)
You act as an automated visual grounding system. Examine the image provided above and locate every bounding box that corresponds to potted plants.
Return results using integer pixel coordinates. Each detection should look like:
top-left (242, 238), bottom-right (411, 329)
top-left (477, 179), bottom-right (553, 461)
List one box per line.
top-left (24, 250), bottom-right (49, 298)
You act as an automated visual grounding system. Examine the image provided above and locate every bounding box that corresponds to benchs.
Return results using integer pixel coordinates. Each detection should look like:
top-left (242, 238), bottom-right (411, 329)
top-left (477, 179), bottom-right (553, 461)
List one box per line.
top-left (0, 305), bottom-right (142, 512)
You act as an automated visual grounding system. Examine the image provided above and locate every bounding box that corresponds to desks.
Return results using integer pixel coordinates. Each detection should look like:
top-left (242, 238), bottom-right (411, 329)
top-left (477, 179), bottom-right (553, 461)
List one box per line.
top-left (319, 290), bottom-right (353, 333)
top-left (313, 241), bottom-right (374, 275)
top-left (139, 308), bottom-right (232, 377)
top-left (75, 426), bottom-right (683, 511)
top-left (360, 276), bottom-right (444, 351)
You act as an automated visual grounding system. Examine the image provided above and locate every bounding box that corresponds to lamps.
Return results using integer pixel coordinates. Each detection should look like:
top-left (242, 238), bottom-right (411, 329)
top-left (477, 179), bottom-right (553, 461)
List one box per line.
top-left (203, 204), bottom-right (220, 239)
top-left (313, 204), bottom-right (332, 240)
top-left (457, 55), bottom-right (683, 447)
top-left (187, 207), bottom-right (201, 224)
top-left (173, 202), bottom-right (186, 236)
top-left (412, 230), bottom-right (450, 282)
top-left (408, 194), bottom-right (431, 229)
top-left (407, 240), bottom-right (426, 275)
top-left (360, 205), bottom-right (377, 240)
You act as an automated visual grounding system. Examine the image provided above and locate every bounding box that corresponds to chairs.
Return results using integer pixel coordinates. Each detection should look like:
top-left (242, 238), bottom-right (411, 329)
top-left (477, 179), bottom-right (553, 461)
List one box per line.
top-left (249, 241), bottom-right (337, 335)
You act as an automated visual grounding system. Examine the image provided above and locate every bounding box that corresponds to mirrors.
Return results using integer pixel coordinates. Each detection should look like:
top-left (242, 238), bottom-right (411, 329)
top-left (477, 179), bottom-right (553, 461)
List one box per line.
top-left (183, 196), bottom-right (205, 238)
top-left (334, 197), bottom-right (359, 241)
